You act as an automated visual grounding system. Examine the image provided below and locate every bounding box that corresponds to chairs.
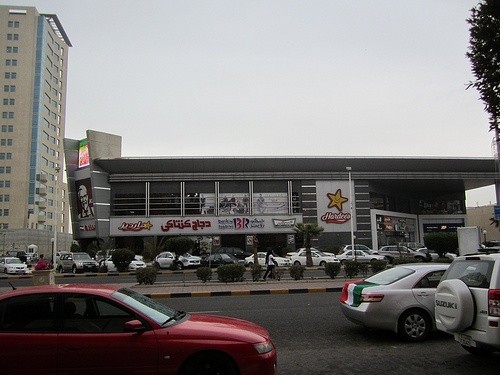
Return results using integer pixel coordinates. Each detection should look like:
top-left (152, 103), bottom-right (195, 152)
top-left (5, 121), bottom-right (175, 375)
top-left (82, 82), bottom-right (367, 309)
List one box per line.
top-left (64, 302), bottom-right (83, 330)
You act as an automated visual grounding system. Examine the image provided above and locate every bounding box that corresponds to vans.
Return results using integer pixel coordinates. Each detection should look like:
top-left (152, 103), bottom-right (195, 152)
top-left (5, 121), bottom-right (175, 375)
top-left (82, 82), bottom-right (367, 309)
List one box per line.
top-left (397, 242), bottom-right (427, 251)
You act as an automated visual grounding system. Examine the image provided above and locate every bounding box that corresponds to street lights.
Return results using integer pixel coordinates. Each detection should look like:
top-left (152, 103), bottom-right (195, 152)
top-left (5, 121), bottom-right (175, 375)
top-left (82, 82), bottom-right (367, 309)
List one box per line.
top-left (52, 166), bottom-right (60, 269)
top-left (484, 229), bottom-right (488, 248)
top-left (346, 166), bottom-right (355, 262)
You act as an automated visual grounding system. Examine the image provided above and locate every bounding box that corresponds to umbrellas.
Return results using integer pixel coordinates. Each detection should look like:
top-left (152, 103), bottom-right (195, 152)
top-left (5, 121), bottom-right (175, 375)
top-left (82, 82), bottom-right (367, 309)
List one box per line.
top-left (35, 261), bottom-right (49, 270)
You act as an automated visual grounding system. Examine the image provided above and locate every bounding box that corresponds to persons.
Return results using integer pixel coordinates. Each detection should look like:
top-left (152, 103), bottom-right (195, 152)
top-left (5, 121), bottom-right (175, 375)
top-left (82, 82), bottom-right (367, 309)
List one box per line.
top-left (39, 254), bottom-right (44, 261)
top-left (263, 249), bottom-right (278, 280)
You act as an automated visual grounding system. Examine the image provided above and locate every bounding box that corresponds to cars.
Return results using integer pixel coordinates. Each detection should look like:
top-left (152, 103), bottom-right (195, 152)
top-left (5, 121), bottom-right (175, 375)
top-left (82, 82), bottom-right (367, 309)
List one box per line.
top-left (0, 257), bottom-right (27, 275)
top-left (417, 247), bottom-right (458, 260)
top-left (152, 251), bottom-right (202, 269)
top-left (1, 284), bottom-right (277, 374)
top-left (101, 255), bottom-right (146, 272)
top-left (340, 262), bottom-right (454, 341)
top-left (335, 250), bottom-right (384, 264)
top-left (244, 252), bottom-right (290, 266)
top-left (286, 247), bottom-right (335, 258)
top-left (200, 253), bottom-right (247, 267)
top-left (290, 251), bottom-right (340, 266)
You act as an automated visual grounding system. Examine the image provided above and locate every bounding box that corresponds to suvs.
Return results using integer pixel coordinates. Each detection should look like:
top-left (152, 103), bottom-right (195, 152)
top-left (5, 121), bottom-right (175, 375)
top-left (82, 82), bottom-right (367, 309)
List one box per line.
top-left (340, 244), bottom-right (378, 254)
top-left (95, 249), bottom-right (144, 263)
top-left (434, 249), bottom-right (500, 357)
top-left (378, 244), bottom-right (426, 261)
top-left (211, 247), bottom-right (252, 259)
top-left (57, 252), bottom-right (99, 274)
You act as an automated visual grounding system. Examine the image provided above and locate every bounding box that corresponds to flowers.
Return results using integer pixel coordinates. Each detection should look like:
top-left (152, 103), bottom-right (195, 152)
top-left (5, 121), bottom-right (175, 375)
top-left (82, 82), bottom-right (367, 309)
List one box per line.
top-left (33, 260), bottom-right (54, 270)
